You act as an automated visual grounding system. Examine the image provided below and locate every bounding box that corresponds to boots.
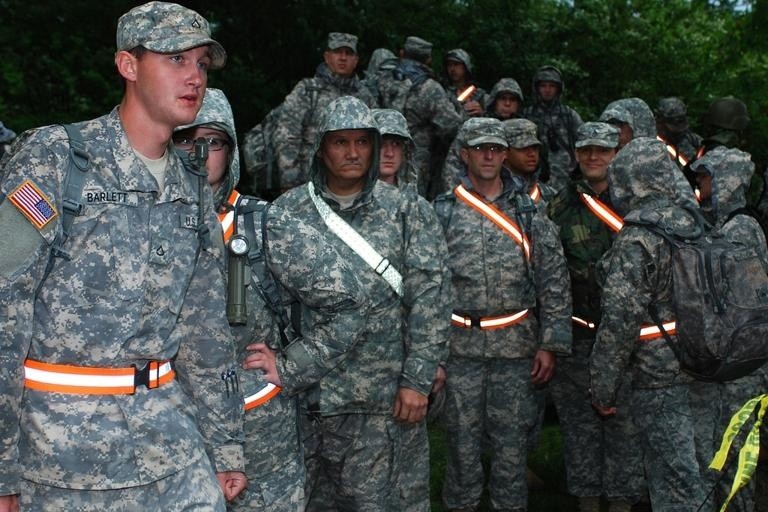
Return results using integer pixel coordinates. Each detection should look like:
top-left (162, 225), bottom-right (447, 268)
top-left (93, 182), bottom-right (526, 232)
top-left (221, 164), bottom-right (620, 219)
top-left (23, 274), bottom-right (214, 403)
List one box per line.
top-left (577, 497), bottom-right (632, 512)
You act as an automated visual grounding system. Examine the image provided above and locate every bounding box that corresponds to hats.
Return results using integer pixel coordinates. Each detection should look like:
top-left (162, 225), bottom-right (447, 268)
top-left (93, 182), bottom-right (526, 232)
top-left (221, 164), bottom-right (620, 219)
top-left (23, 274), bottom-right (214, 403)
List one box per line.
top-left (502, 118), bottom-right (543, 150)
top-left (116, 0), bottom-right (228, 71)
top-left (326, 32), bottom-right (359, 53)
top-left (456, 117), bottom-right (509, 149)
top-left (403, 36), bottom-right (434, 57)
top-left (573, 120), bottom-right (621, 149)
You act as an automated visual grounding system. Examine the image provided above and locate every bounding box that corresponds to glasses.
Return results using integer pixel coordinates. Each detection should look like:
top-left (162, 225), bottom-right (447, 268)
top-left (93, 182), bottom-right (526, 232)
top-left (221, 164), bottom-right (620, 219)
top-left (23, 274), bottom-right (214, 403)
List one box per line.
top-left (173, 137), bottom-right (231, 152)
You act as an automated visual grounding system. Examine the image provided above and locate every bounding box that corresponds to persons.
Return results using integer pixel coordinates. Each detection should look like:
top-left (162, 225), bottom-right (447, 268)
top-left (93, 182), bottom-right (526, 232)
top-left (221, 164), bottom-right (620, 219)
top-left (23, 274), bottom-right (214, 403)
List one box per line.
top-left (683, 144), bottom-right (768, 512)
top-left (272, 95), bottom-right (455, 512)
top-left (426, 114), bottom-right (572, 512)
top-left (590, 136), bottom-right (718, 512)
top-left (540, 119), bottom-right (648, 512)
top-left (171, 87), bottom-right (367, 512)
top-left (1, 2), bottom-right (249, 512)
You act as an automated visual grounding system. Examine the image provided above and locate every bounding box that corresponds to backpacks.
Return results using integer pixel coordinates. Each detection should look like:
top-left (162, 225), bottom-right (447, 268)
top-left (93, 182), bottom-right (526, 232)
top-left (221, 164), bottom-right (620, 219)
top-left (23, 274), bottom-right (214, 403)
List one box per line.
top-left (651, 202), bottom-right (767, 383)
top-left (242, 101), bottom-right (281, 191)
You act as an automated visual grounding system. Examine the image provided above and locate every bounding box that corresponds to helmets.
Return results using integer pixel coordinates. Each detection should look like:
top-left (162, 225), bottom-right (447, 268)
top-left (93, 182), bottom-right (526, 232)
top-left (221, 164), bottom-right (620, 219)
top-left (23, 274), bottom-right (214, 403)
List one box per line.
top-left (705, 98), bottom-right (751, 131)
